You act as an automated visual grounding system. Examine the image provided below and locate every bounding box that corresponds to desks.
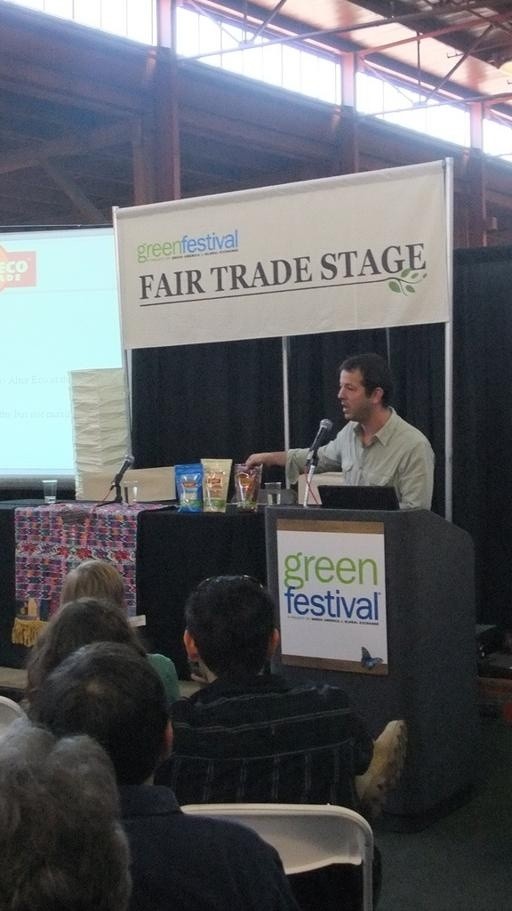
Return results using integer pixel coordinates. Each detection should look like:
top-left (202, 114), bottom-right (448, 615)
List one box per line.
top-left (1, 497), bottom-right (263, 684)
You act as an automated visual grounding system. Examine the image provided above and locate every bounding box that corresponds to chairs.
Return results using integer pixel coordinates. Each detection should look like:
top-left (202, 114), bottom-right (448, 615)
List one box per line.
top-left (178, 804), bottom-right (376, 911)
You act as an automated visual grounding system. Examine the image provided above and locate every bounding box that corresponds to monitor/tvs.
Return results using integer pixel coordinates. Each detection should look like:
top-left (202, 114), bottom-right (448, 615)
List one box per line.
top-left (316, 482), bottom-right (400, 510)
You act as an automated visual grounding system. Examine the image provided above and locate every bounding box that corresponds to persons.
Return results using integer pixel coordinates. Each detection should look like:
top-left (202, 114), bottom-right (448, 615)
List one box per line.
top-left (0, 721), bottom-right (132, 911)
top-left (149, 571), bottom-right (410, 811)
top-left (58, 560), bottom-right (182, 705)
top-left (16, 604), bottom-right (142, 726)
top-left (30, 641), bottom-right (305, 911)
top-left (243, 350), bottom-right (436, 512)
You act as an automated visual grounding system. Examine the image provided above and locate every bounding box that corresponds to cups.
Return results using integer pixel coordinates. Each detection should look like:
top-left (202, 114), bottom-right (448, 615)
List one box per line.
top-left (41, 479), bottom-right (58, 504)
top-left (123, 479), bottom-right (138, 509)
top-left (264, 482), bottom-right (282, 507)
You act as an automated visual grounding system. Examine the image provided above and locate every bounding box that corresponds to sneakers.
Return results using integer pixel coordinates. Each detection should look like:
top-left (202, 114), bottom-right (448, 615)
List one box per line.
top-left (353, 718), bottom-right (411, 831)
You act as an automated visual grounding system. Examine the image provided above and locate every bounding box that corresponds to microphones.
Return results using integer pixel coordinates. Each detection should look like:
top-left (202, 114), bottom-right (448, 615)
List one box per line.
top-left (303, 418), bottom-right (333, 460)
top-left (110, 454), bottom-right (136, 489)
